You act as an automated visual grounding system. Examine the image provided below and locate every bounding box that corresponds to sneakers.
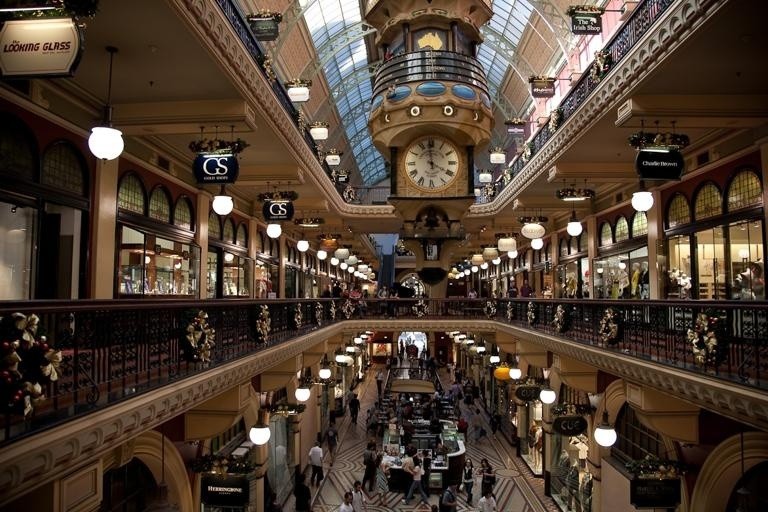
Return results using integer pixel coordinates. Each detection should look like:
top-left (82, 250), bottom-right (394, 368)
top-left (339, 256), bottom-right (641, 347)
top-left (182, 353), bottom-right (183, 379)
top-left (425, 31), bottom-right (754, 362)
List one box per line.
top-left (400, 493), bottom-right (430, 505)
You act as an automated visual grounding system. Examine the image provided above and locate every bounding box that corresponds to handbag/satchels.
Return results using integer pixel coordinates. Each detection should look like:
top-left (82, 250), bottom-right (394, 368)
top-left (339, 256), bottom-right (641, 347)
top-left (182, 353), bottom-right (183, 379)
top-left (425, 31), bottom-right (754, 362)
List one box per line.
top-left (368, 453), bottom-right (380, 469)
top-left (459, 481), bottom-right (466, 491)
top-left (480, 428), bottom-right (487, 438)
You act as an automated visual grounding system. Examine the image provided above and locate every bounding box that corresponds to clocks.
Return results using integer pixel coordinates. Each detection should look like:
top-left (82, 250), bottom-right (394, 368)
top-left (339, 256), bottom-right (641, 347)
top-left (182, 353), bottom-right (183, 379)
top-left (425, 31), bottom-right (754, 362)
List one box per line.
top-left (403, 136), bottom-right (466, 192)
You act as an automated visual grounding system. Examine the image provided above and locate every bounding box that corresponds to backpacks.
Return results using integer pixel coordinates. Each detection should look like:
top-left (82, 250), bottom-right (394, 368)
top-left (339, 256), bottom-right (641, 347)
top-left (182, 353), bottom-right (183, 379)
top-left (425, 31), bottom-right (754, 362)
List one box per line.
top-left (439, 489), bottom-right (455, 512)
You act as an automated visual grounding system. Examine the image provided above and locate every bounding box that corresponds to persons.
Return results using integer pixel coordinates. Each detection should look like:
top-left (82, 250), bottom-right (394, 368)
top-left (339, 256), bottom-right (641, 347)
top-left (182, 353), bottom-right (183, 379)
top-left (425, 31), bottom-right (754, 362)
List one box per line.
top-left (565, 261), bottom-right (650, 324)
top-left (289, 338), bottom-right (500, 512)
top-left (321, 279), bottom-right (534, 320)
top-left (527, 419), bottom-right (593, 512)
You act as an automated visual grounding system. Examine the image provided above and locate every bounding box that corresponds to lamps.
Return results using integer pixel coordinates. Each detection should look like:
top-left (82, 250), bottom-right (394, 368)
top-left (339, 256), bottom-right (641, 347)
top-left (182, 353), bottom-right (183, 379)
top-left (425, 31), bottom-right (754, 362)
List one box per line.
top-left (446, 177), bottom-right (656, 279)
top-left (247, 329), bottom-right (374, 447)
top-left (88, 45), bottom-right (126, 162)
top-left (212, 185), bottom-right (236, 217)
top-left (444, 328), bottom-right (618, 448)
top-left (266, 220), bottom-right (375, 284)
